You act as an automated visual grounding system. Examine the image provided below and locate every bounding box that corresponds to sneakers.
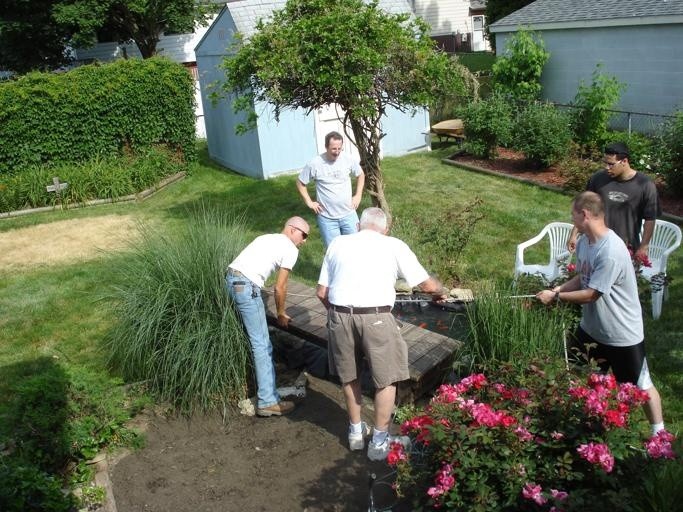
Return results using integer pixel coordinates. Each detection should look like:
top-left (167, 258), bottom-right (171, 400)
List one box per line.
top-left (347, 419), bottom-right (371, 452)
top-left (257, 399), bottom-right (296, 418)
top-left (367, 434), bottom-right (411, 463)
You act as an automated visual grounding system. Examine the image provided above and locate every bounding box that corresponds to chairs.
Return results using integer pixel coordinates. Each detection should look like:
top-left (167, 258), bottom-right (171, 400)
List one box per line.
top-left (636, 220), bottom-right (682, 320)
top-left (511, 221), bottom-right (576, 289)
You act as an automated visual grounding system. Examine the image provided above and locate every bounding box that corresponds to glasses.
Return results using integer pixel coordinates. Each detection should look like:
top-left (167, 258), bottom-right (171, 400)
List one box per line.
top-left (601, 159), bottom-right (622, 168)
top-left (289, 224), bottom-right (308, 240)
top-left (328, 147), bottom-right (343, 150)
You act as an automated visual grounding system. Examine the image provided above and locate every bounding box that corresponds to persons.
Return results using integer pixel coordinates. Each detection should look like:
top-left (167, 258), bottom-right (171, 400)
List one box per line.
top-left (295, 131), bottom-right (365, 251)
top-left (566, 141), bottom-right (659, 273)
top-left (535, 191), bottom-right (665, 445)
top-left (225, 215), bottom-right (310, 418)
top-left (315, 207), bottom-right (450, 463)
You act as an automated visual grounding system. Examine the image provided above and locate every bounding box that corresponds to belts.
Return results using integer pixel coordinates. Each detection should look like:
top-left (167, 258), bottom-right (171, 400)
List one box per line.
top-left (324, 297), bottom-right (391, 315)
top-left (226, 265), bottom-right (243, 277)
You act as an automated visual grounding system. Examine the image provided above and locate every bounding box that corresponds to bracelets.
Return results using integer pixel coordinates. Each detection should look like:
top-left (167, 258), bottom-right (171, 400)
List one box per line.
top-left (553, 291), bottom-right (562, 304)
